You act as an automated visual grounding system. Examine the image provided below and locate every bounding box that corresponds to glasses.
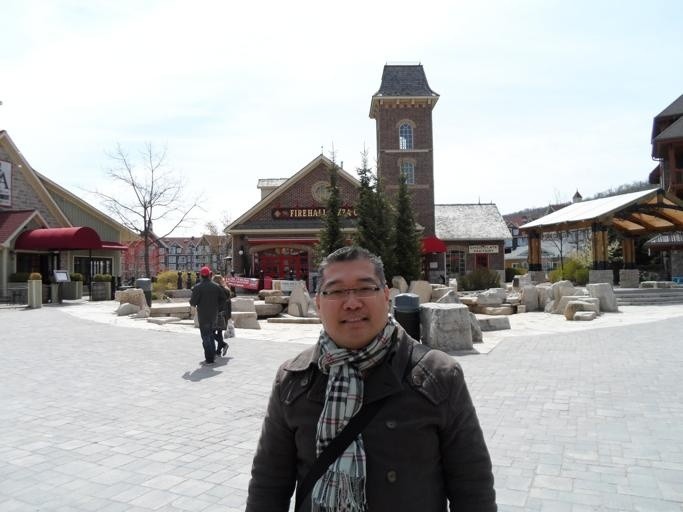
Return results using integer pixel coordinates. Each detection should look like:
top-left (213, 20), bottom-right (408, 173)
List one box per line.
top-left (321, 282), bottom-right (385, 302)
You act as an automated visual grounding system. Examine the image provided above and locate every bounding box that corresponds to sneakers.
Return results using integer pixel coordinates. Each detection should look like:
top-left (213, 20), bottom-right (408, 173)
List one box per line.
top-left (200, 344), bottom-right (228, 367)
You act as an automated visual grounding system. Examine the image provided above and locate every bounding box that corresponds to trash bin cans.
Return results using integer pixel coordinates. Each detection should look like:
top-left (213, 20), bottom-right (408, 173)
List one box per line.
top-left (135, 278), bottom-right (151, 308)
top-left (393, 293), bottom-right (420, 343)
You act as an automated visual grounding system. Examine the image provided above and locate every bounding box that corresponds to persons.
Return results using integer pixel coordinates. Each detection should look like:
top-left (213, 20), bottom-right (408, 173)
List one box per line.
top-left (189, 267), bottom-right (226, 364)
top-left (245, 245), bottom-right (497, 512)
top-left (212, 274), bottom-right (231, 356)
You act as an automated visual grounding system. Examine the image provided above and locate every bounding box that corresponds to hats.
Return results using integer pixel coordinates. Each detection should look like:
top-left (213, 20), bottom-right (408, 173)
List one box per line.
top-left (200, 266), bottom-right (210, 275)
